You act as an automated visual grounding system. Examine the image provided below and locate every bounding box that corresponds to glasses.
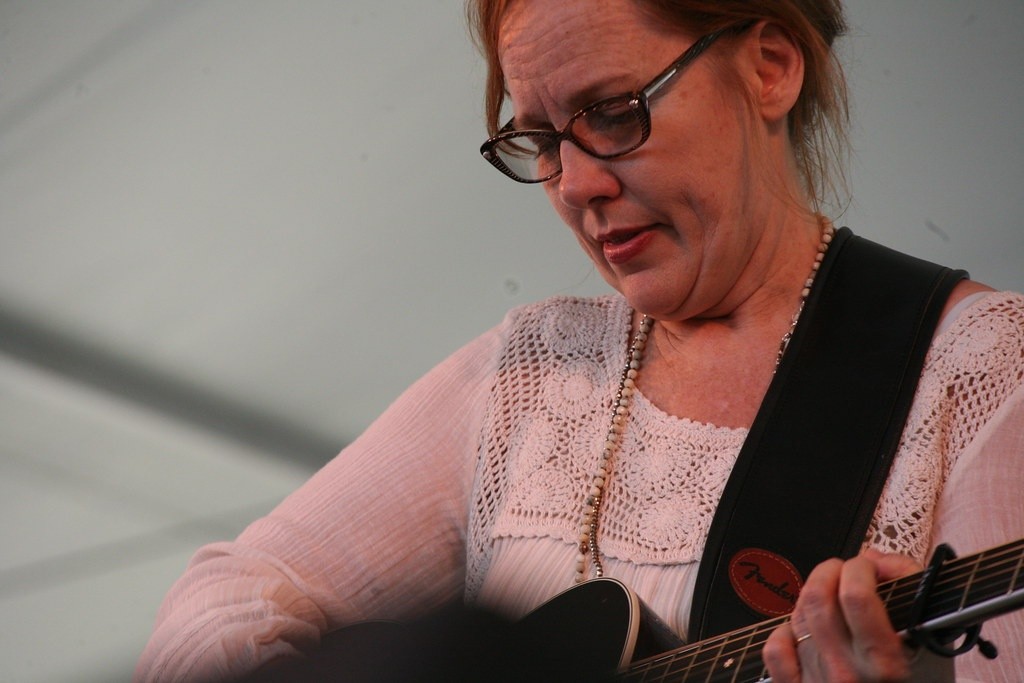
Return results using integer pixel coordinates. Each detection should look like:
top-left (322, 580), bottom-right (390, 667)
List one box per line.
top-left (480, 28), bottom-right (727, 185)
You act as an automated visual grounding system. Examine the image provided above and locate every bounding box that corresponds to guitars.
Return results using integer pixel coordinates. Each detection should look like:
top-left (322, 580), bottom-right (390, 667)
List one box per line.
top-left (298, 534), bottom-right (1023, 683)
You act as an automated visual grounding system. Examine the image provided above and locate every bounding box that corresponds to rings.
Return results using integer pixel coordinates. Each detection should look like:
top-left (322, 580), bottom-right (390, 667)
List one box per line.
top-left (796, 634), bottom-right (812, 642)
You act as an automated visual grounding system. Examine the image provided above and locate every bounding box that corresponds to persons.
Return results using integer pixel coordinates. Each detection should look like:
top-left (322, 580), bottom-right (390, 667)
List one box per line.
top-left (136, 0), bottom-right (1024, 683)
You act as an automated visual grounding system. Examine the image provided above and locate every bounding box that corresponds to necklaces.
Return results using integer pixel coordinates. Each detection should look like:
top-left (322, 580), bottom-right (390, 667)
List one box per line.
top-left (575, 213), bottom-right (832, 586)
